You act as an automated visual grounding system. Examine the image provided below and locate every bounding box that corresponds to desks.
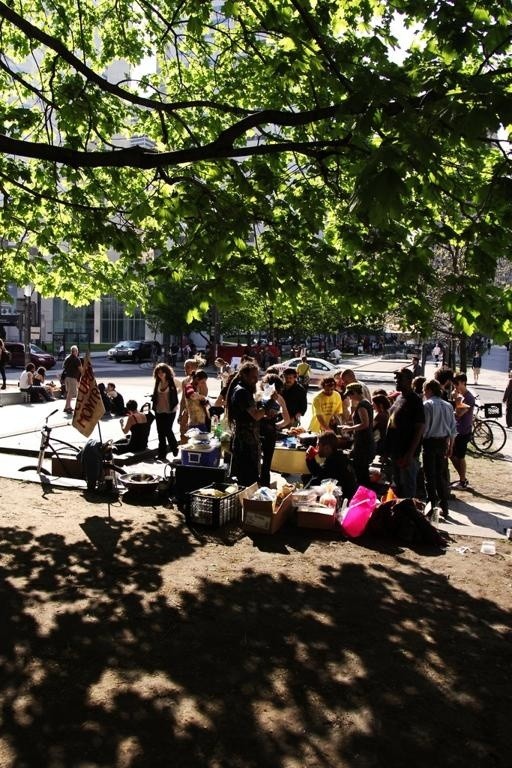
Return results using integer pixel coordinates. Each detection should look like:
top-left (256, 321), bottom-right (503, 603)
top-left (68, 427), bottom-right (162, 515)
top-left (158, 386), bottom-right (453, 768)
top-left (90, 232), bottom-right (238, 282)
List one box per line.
top-left (23, 284), bottom-right (32, 367)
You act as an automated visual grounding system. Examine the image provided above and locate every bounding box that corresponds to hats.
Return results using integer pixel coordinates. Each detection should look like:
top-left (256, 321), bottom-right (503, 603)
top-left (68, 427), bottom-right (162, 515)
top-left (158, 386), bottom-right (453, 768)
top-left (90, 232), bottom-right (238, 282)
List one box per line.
top-left (107, 339), bottom-right (161, 362)
top-left (2, 340), bottom-right (55, 369)
top-left (268, 358), bottom-right (348, 389)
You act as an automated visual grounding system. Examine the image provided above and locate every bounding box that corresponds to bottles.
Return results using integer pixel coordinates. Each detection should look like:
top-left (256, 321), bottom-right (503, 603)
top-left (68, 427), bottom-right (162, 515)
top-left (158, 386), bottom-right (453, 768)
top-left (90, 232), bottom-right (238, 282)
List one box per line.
top-left (395, 368), bottom-right (415, 380)
top-left (344, 383), bottom-right (362, 396)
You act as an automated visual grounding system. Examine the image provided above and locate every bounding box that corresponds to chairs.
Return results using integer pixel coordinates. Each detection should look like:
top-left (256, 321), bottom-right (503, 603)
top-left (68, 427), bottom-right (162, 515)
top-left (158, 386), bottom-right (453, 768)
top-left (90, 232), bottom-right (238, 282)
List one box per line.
top-left (63, 407), bottom-right (74, 413)
top-left (449, 480), bottom-right (469, 491)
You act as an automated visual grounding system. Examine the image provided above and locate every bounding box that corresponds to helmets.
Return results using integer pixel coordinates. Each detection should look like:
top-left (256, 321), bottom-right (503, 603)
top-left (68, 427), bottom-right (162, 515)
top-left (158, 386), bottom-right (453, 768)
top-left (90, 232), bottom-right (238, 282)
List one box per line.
top-left (336, 426), bottom-right (349, 428)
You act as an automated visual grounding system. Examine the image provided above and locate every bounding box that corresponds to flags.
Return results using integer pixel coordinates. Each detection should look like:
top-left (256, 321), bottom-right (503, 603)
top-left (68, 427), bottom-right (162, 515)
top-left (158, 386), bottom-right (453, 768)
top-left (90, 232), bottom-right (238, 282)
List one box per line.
top-left (71, 346), bottom-right (105, 438)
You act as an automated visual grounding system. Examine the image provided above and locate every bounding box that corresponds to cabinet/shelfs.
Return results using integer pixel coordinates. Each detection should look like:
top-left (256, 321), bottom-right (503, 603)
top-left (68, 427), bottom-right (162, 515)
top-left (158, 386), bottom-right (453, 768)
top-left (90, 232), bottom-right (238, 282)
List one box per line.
top-left (262, 384), bottom-right (271, 402)
top-left (211, 416), bottom-right (222, 438)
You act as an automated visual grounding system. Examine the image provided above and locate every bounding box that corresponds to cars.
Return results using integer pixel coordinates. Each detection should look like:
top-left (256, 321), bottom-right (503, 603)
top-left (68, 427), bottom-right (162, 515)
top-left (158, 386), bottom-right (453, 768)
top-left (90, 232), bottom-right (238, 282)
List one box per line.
top-left (308, 446), bottom-right (319, 457)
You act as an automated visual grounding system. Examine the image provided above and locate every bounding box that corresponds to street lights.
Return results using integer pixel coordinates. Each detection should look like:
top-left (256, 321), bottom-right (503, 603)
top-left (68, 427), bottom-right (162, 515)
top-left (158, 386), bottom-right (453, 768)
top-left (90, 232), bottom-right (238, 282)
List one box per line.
top-left (298, 375), bottom-right (305, 381)
top-left (1, 349), bottom-right (12, 363)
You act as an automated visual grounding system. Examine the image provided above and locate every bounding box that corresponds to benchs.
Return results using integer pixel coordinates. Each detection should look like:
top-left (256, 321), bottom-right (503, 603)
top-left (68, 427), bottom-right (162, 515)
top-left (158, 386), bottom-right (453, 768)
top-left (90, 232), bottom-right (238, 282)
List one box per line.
top-left (71, 346), bottom-right (105, 438)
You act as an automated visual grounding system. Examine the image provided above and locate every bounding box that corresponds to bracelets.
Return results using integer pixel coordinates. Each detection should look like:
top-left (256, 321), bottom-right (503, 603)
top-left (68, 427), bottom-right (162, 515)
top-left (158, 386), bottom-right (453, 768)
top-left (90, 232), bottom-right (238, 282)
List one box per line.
top-left (275, 441), bottom-right (284, 447)
top-left (118, 472), bottom-right (163, 494)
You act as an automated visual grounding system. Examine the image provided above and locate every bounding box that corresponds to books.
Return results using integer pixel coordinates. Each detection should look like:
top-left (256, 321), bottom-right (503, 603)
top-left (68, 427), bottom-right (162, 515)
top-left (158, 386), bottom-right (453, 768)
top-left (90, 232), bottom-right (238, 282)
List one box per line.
top-left (297, 505), bottom-right (336, 532)
top-left (270, 446), bottom-right (326, 475)
top-left (179, 441), bottom-right (222, 467)
top-left (52, 450), bottom-right (113, 481)
top-left (238, 482), bottom-right (293, 533)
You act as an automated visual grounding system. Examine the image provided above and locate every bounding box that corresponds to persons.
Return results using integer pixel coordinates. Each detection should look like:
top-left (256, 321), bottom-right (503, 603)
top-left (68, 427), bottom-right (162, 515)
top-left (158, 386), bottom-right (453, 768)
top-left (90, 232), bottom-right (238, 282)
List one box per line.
top-left (18, 362), bottom-right (54, 401)
top-left (177, 353), bottom-right (312, 497)
top-left (62, 345), bottom-right (82, 413)
top-left (331, 346), bottom-right (342, 364)
top-left (152, 335), bottom-right (211, 369)
top-left (30, 367), bottom-right (45, 403)
top-left (503, 370), bottom-right (512, 427)
top-left (486, 339), bottom-right (493, 355)
top-left (111, 400), bottom-right (150, 453)
top-left (432, 344), bottom-right (443, 366)
top-left (106, 383), bottom-right (124, 414)
top-left (472, 351), bottom-right (480, 385)
top-left (97, 383), bottom-right (114, 420)
top-left (151, 362), bottom-right (183, 462)
top-left (249, 343), bottom-right (307, 371)
top-left (0, 337), bottom-right (8, 389)
top-left (411, 357), bottom-right (424, 378)
top-left (369, 340), bottom-right (385, 357)
top-left (305, 359), bottom-right (475, 516)
